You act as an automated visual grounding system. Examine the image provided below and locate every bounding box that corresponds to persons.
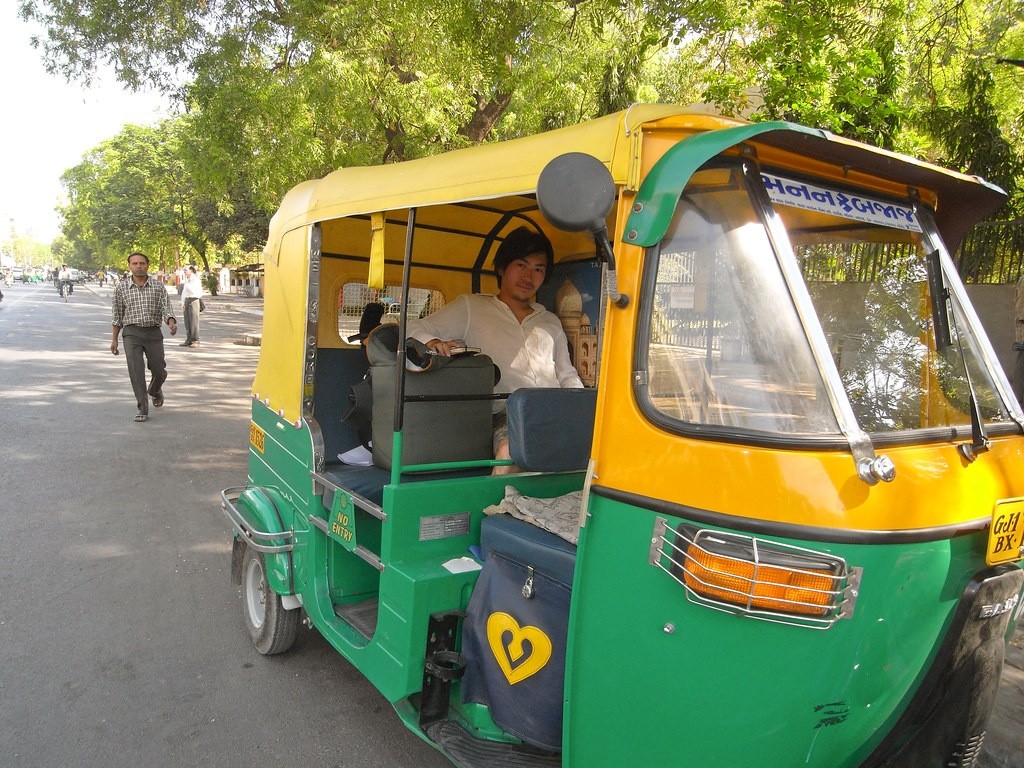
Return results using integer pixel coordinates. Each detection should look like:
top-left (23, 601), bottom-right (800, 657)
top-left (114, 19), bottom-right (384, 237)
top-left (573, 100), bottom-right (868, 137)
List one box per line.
top-left (109, 252), bottom-right (177, 422)
top-left (96, 269), bottom-right (105, 287)
top-left (58, 265), bottom-right (73, 297)
top-left (53, 268), bottom-right (59, 286)
top-left (405, 225), bottom-right (585, 476)
top-left (175, 265), bottom-right (203, 347)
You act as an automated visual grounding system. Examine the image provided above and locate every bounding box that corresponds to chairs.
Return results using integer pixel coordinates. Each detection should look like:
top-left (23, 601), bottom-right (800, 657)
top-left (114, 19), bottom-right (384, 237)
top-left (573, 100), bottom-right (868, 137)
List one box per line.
top-left (480, 387), bottom-right (598, 590)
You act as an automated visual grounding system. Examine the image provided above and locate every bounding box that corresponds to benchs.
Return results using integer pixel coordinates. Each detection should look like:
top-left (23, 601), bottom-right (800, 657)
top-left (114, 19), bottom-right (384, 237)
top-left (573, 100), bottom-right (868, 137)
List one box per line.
top-left (315, 348), bottom-right (501, 510)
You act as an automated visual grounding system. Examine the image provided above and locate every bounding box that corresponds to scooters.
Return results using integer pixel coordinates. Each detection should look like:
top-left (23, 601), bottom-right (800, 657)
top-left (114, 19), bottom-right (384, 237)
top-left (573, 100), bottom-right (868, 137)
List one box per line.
top-left (97, 274), bottom-right (104, 287)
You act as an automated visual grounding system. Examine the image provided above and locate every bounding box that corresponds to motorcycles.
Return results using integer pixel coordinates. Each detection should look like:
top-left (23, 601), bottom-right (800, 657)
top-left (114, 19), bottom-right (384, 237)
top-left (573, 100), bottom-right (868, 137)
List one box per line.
top-left (221, 102), bottom-right (1023, 768)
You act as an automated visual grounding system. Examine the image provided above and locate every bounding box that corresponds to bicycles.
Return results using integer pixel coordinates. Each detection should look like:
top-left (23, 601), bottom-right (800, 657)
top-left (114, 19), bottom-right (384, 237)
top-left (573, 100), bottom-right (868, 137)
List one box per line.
top-left (60, 279), bottom-right (71, 302)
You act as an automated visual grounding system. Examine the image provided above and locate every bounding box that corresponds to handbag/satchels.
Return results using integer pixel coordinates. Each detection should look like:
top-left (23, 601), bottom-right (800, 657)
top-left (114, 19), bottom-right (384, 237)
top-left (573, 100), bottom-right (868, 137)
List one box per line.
top-left (200, 298), bottom-right (206, 312)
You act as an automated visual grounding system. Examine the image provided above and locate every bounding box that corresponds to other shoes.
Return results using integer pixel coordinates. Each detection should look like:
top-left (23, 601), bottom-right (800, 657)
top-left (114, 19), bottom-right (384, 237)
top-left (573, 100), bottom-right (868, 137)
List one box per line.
top-left (152, 392), bottom-right (164, 408)
top-left (179, 342), bottom-right (191, 346)
top-left (190, 343), bottom-right (200, 348)
top-left (134, 414), bottom-right (149, 422)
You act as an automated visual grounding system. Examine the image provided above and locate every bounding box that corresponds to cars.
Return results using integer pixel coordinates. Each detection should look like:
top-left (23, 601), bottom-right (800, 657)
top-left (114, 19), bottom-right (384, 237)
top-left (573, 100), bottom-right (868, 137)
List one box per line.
top-left (0, 266), bottom-right (87, 288)
